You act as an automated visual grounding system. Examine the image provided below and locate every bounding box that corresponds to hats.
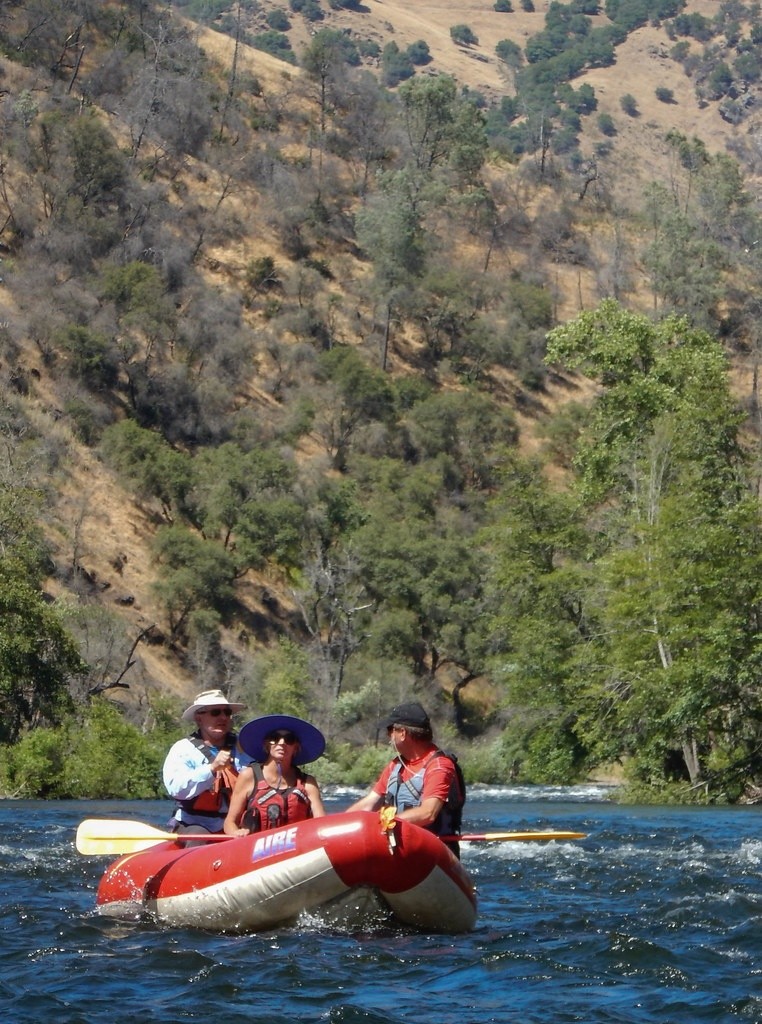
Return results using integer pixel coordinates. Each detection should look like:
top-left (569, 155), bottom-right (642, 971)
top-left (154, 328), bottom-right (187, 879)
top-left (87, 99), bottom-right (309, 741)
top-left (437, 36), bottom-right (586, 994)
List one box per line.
top-left (239, 715), bottom-right (326, 766)
top-left (180, 689), bottom-right (245, 721)
top-left (375, 703), bottom-right (430, 729)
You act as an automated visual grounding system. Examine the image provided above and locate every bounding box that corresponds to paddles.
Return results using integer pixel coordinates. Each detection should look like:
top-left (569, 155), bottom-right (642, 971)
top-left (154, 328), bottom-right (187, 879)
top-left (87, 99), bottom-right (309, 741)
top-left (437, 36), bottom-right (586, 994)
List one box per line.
top-left (73, 816), bottom-right (236, 856)
top-left (435, 830), bottom-right (588, 842)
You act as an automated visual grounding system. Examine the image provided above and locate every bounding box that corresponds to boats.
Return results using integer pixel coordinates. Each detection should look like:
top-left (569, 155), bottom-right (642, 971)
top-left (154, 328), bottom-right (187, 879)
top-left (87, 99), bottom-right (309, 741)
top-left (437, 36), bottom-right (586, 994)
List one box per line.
top-left (96, 806), bottom-right (477, 933)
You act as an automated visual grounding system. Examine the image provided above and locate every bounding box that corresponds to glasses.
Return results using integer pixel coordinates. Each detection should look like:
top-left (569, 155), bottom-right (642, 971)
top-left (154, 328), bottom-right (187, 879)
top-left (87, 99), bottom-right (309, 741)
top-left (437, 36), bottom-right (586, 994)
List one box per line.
top-left (267, 732), bottom-right (299, 745)
top-left (201, 707), bottom-right (233, 717)
top-left (386, 725), bottom-right (400, 732)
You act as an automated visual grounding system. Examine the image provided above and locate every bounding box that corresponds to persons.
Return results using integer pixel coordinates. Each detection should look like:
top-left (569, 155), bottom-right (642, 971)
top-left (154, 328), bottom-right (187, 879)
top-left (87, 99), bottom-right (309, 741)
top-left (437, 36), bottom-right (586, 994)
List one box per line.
top-left (223, 715), bottom-right (328, 840)
top-left (163, 690), bottom-right (255, 849)
top-left (344, 702), bottom-right (461, 861)
